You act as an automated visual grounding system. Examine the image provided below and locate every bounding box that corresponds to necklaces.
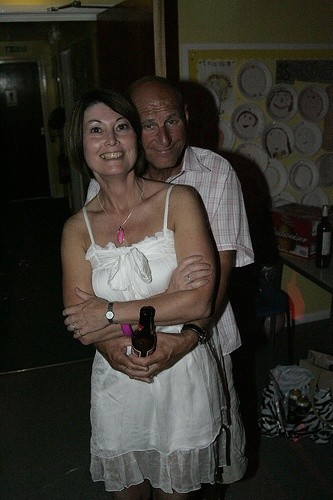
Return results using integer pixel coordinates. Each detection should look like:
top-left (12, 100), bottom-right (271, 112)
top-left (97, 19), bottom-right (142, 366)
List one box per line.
top-left (98, 178), bottom-right (144, 244)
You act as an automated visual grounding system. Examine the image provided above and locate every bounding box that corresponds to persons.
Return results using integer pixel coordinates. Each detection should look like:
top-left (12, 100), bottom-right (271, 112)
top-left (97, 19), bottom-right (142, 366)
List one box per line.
top-left (61, 88), bottom-right (227, 500)
top-left (95, 76), bottom-right (255, 500)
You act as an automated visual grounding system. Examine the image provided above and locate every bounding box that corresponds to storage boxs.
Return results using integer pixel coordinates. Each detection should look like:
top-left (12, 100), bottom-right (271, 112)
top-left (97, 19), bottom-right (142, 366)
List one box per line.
top-left (273, 201), bottom-right (323, 261)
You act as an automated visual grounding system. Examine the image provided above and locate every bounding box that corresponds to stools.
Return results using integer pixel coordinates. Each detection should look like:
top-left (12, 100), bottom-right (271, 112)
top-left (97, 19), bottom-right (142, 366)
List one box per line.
top-left (246, 287), bottom-right (292, 356)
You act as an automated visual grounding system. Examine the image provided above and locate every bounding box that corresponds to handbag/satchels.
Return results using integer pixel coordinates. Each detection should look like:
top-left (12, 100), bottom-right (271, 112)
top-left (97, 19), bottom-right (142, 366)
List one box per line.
top-left (252, 372), bottom-right (333, 445)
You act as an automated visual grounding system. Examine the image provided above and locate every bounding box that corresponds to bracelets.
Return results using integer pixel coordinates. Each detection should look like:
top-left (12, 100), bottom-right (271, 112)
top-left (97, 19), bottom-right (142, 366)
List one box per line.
top-left (121, 324), bottom-right (133, 337)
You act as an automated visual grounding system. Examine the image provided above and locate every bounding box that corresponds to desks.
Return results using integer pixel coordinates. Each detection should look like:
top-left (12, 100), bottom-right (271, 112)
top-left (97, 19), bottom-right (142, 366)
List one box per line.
top-left (278, 250), bottom-right (333, 327)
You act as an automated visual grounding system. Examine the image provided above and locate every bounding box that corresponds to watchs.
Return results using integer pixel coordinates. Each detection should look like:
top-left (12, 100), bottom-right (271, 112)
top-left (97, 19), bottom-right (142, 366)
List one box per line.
top-left (105, 302), bottom-right (114, 323)
top-left (182, 324), bottom-right (207, 345)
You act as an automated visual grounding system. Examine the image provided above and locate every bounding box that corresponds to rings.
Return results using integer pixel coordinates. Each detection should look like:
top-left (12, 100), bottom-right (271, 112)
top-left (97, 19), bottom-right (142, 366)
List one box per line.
top-left (79, 330), bottom-right (83, 336)
top-left (73, 324), bottom-right (77, 330)
top-left (188, 276), bottom-right (191, 281)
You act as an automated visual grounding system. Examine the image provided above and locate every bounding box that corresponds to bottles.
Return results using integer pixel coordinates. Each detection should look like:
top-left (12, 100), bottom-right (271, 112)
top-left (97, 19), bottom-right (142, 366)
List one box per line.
top-left (288, 389), bottom-right (302, 424)
top-left (316, 206), bottom-right (333, 269)
top-left (132, 306), bottom-right (157, 358)
top-left (296, 395), bottom-right (310, 425)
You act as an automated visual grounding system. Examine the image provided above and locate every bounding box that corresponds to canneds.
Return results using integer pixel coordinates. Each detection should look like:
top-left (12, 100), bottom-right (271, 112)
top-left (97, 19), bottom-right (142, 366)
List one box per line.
top-left (286, 389), bottom-right (310, 427)
